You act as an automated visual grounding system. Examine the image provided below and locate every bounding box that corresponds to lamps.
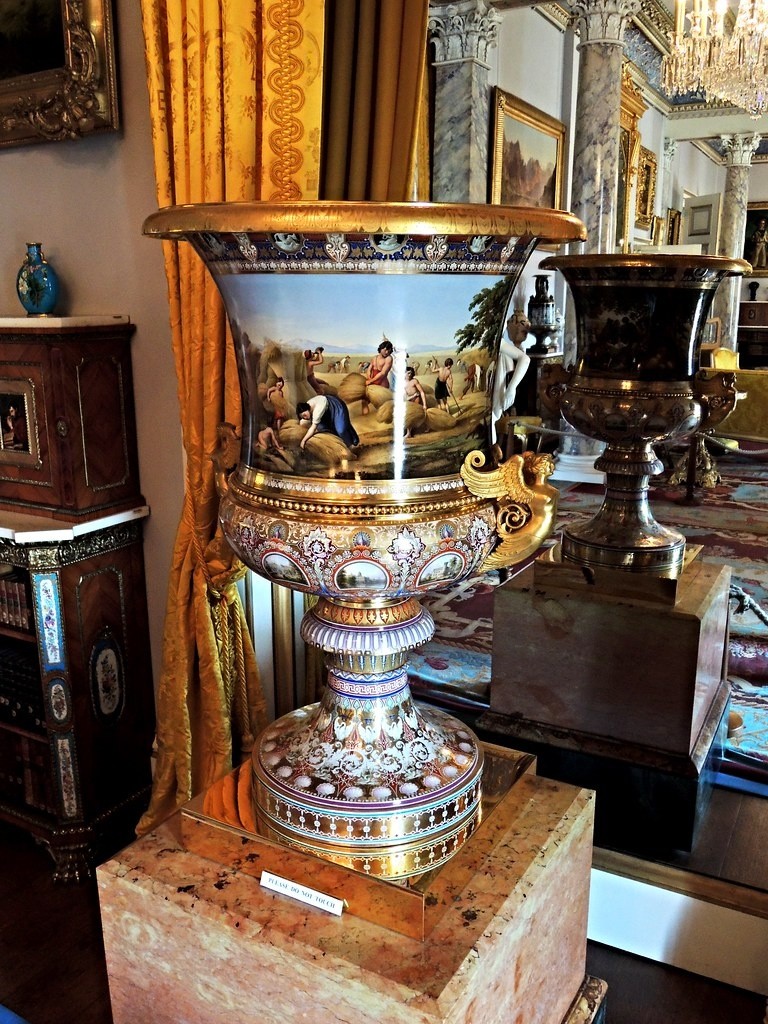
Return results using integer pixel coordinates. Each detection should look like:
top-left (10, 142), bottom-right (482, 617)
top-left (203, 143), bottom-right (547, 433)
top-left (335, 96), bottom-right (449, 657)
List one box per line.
top-left (659, 0), bottom-right (768, 120)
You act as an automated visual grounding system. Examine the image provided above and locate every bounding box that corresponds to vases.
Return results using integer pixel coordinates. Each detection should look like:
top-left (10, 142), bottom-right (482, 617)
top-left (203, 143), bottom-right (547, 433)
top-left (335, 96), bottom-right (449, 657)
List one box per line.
top-left (14, 242), bottom-right (59, 318)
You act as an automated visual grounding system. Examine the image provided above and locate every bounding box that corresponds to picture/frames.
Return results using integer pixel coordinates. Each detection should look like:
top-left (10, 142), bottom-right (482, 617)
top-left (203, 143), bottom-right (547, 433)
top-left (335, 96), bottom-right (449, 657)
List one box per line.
top-left (491, 85), bottom-right (565, 251)
top-left (742, 201), bottom-right (768, 278)
